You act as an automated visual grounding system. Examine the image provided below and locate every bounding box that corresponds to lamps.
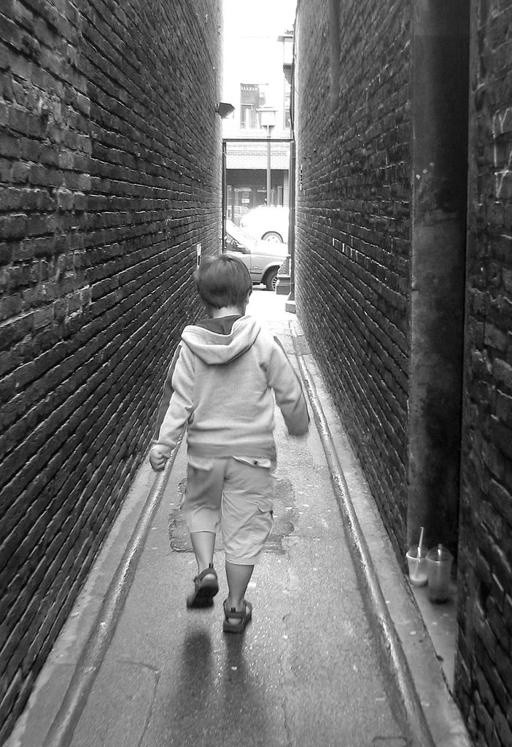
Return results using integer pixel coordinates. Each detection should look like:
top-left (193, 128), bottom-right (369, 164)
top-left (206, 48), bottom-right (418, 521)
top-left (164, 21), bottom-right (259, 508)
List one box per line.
top-left (216, 102), bottom-right (235, 119)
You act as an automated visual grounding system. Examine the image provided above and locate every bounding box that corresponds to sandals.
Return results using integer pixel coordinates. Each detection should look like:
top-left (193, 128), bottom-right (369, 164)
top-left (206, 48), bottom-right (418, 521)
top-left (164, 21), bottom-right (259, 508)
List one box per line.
top-left (223, 598), bottom-right (252, 633)
top-left (186, 562), bottom-right (219, 609)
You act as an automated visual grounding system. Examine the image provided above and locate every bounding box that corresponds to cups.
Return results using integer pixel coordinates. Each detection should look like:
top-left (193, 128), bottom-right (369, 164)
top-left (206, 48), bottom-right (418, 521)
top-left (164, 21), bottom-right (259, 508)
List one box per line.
top-left (425, 543), bottom-right (454, 605)
top-left (406, 544), bottom-right (429, 587)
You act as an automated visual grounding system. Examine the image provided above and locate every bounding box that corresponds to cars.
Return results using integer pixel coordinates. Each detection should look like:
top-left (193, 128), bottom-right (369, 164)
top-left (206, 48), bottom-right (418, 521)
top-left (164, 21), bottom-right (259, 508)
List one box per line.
top-left (226, 206), bottom-right (289, 290)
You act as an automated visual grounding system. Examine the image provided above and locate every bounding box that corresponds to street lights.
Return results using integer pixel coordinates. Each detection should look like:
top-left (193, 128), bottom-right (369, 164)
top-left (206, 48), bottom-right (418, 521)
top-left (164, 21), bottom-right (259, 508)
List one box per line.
top-left (256, 105), bottom-right (278, 206)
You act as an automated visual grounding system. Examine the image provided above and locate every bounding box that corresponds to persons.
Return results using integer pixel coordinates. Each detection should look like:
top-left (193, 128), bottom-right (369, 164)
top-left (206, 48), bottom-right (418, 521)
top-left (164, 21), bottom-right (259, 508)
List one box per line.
top-left (149, 252), bottom-right (310, 635)
